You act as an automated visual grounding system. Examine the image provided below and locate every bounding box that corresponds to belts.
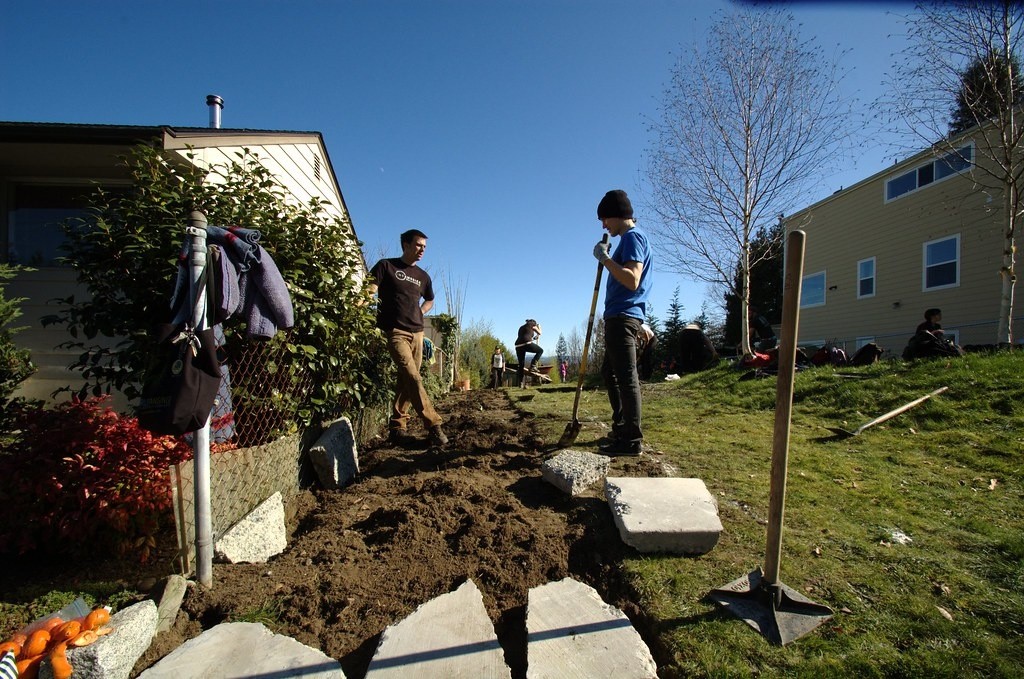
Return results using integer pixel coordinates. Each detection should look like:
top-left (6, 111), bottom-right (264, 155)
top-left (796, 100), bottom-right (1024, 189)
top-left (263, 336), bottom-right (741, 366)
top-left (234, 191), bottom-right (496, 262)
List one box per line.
top-left (515, 342), bottom-right (531, 347)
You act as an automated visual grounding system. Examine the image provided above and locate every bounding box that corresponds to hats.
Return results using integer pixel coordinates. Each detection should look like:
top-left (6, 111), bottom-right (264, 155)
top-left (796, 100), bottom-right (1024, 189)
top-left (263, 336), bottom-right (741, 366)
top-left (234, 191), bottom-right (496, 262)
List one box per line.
top-left (495, 346), bottom-right (500, 349)
top-left (597, 190), bottom-right (633, 219)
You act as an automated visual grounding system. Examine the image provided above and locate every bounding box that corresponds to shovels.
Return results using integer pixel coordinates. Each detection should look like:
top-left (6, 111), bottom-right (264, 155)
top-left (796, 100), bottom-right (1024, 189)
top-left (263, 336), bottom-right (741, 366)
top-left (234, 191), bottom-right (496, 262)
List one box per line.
top-left (558, 233), bottom-right (609, 444)
top-left (532, 323), bottom-right (542, 386)
top-left (826, 386), bottom-right (948, 437)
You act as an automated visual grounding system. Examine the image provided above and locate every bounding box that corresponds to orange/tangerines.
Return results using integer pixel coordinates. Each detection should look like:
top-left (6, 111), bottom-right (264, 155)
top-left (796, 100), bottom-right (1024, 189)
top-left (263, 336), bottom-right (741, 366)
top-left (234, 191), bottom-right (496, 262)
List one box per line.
top-left (0, 609), bottom-right (88, 679)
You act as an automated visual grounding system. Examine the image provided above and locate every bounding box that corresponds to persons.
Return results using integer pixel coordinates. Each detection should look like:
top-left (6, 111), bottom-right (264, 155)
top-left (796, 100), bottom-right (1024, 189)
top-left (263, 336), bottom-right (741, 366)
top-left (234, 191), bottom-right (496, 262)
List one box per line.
top-left (491, 345), bottom-right (506, 391)
top-left (737, 306), bottom-right (777, 353)
top-left (514, 319), bottom-right (544, 390)
top-left (560, 360), bottom-right (569, 383)
top-left (354, 229), bottom-right (449, 446)
top-left (915, 308), bottom-right (946, 344)
top-left (593, 189), bottom-right (653, 456)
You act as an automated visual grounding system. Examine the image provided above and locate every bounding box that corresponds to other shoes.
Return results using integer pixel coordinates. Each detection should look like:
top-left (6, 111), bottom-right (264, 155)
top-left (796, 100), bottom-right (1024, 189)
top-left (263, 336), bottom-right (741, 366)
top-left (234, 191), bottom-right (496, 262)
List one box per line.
top-left (519, 383), bottom-right (524, 388)
top-left (529, 366), bottom-right (540, 373)
top-left (391, 429), bottom-right (405, 438)
top-left (428, 425), bottom-right (449, 445)
top-left (599, 430), bottom-right (642, 455)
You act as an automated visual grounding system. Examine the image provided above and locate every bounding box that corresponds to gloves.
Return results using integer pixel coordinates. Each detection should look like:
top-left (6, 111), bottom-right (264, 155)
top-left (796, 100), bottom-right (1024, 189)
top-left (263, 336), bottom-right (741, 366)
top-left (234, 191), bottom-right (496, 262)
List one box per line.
top-left (593, 241), bottom-right (611, 265)
